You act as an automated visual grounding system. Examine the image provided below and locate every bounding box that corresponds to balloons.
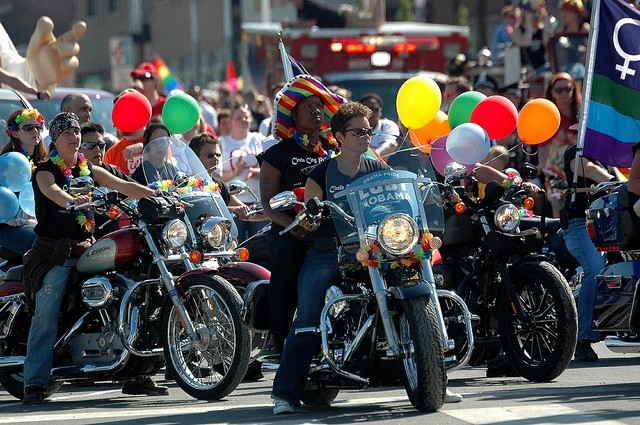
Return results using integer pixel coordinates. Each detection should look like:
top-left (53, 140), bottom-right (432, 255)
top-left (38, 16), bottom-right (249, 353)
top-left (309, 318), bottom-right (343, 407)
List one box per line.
top-left (447, 91), bottom-right (488, 130)
top-left (0, 151), bottom-right (31, 192)
top-left (396, 75), bottom-right (442, 130)
top-left (18, 179), bottom-right (37, 219)
top-left (111, 91), bottom-right (152, 133)
top-left (431, 136), bottom-right (476, 178)
top-left (517, 97), bottom-right (561, 145)
top-left (409, 110), bottom-right (452, 155)
top-left (162, 94), bottom-right (201, 135)
top-left (446, 123), bottom-right (490, 165)
top-left (0, 188), bottom-right (19, 222)
top-left (469, 95), bottom-right (519, 141)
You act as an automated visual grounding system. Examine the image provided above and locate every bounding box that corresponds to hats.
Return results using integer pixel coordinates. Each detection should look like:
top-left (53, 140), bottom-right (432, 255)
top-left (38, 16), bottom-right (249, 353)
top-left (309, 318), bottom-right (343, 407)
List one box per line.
top-left (271, 75), bottom-right (348, 142)
top-left (131, 62), bottom-right (158, 77)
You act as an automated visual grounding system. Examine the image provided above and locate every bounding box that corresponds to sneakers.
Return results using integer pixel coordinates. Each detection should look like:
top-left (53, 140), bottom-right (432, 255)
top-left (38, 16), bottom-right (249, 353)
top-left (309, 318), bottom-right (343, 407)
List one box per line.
top-left (444, 388), bottom-right (464, 402)
top-left (165, 363), bottom-right (212, 378)
top-left (574, 347), bottom-right (598, 361)
top-left (122, 377), bottom-right (168, 395)
top-left (272, 400), bottom-right (292, 414)
top-left (22, 387), bottom-right (42, 403)
top-left (487, 364), bottom-right (521, 377)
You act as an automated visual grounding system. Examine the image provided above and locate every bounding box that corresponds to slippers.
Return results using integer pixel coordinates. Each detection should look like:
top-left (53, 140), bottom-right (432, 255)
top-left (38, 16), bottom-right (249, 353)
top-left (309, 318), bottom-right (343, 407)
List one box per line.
top-left (259, 342), bottom-right (282, 356)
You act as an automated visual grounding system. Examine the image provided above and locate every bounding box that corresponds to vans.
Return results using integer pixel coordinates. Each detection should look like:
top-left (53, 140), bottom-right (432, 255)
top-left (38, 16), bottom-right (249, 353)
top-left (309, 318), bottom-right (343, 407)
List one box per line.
top-left (19, 88), bottom-right (118, 148)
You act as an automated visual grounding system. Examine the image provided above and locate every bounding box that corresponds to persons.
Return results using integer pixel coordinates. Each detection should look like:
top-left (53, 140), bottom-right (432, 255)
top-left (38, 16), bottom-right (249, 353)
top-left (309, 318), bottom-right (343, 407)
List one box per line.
top-left (479, 145), bottom-right (509, 171)
top-left (561, 105), bottom-right (629, 361)
top-left (77, 122), bottom-right (142, 196)
top-left (22, 112), bottom-right (195, 404)
top-left (542, 0), bottom-right (590, 48)
top-left (556, 36), bottom-right (585, 79)
top-left (102, 88), bottom-right (147, 176)
top-left (386, 118), bottom-right (540, 287)
top-left (187, 132), bottom-right (250, 220)
top-left (258, 82), bottom-right (285, 136)
top-left (469, 49), bottom-right (498, 91)
top-left (131, 62), bottom-right (166, 114)
top-left (0, 16), bottom-right (87, 98)
top-left (131, 124), bottom-right (200, 192)
top-left (43, 92), bottom-right (93, 146)
top-left (217, 109), bottom-right (231, 136)
top-left (213, 104), bottom-right (269, 249)
top-left (255, 95), bottom-right (349, 350)
top-left (446, 54), bottom-right (477, 92)
top-left (535, 71), bottom-right (582, 218)
top-left (441, 81), bottom-right (459, 113)
top-left (271, 100), bottom-right (463, 414)
top-left (359, 94), bottom-right (401, 157)
top-left (490, 5), bottom-right (533, 66)
top-left (474, 84), bottom-right (494, 97)
top-left (0, 109), bottom-right (48, 256)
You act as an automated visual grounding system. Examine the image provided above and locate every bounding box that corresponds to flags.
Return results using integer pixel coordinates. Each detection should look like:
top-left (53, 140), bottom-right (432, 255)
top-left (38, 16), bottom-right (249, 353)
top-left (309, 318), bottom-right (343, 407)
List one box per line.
top-left (571, 0), bottom-right (640, 202)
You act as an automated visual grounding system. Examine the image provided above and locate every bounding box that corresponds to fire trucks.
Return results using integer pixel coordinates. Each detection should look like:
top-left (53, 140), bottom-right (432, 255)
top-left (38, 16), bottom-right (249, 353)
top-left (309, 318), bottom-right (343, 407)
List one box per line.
top-left (238, 18), bottom-right (473, 134)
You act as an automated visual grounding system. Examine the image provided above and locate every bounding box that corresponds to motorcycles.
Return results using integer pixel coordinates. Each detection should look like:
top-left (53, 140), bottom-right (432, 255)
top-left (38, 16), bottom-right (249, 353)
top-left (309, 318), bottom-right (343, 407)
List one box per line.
top-left (1, 136), bottom-right (252, 402)
top-left (585, 179), bottom-right (640, 356)
top-left (426, 161), bottom-right (582, 382)
top-left (514, 178), bottom-right (640, 344)
top-left (240, 132), bottom-right (476, 416)
top-left (142, 138), bottom-right (273, 384)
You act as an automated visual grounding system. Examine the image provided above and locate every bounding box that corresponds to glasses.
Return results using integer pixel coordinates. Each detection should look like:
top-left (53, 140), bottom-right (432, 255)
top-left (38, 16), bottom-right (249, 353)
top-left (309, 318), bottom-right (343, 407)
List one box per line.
top-left (345, 128), bottom-right (372, 137)
top-left (81, 141), bottom-right (106, 150)
top-left (231, 103), bottom-right (249, 110)
top-left (207, 151), bottom-right (221, 158)
top-left (366, 106), bottom-right (382, 113)
top-left (556, 86), bottom-right (572, 93)
top-left (17, 123), bottom-right (43, 132)
